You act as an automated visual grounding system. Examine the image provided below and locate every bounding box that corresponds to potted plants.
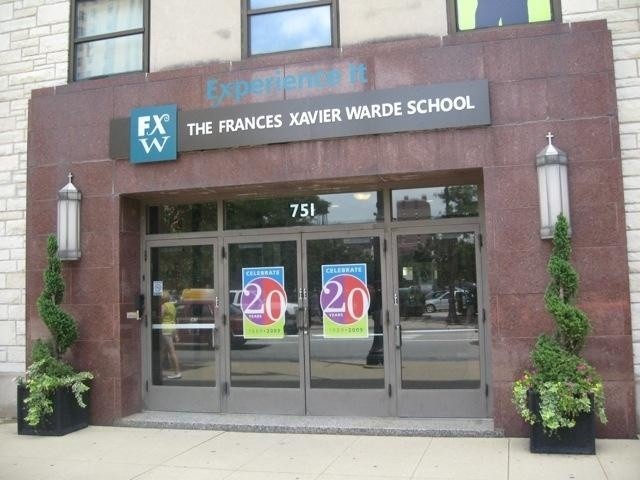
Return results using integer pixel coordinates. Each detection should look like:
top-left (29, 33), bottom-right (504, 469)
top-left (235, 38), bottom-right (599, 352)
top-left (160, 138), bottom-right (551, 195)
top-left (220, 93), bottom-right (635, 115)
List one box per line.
top-left (15, 234), bottom-right (95, 436)
top-left (513, 212), bottom-right (609, 455)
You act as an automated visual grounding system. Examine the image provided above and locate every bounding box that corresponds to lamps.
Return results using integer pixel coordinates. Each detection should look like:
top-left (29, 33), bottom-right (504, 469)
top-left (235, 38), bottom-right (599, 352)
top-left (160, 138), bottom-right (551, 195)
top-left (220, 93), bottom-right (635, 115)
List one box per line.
top-left (55, 173), bottom-right (81, 263)
top-left (537, 131), bottom-right (573, 240)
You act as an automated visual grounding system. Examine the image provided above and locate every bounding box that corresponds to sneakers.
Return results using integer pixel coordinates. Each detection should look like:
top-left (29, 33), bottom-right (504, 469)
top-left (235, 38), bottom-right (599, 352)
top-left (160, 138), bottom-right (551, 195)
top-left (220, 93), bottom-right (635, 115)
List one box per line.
top-left (167, 373), bottom-right (181, 379)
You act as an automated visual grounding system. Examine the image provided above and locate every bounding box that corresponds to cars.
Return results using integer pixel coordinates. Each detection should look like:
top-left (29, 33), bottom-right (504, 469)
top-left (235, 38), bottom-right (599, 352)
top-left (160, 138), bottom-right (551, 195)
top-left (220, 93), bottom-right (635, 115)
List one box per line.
top-left (399, 288), bottom-right (465, 316)
top-left (174, 289), bottom-right (298, 350)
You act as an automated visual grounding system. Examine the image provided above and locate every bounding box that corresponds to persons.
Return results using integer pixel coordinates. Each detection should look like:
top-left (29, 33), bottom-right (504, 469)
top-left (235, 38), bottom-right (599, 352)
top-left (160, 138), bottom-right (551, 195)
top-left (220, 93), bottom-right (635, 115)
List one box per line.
top-left (157, 289), bottom-right (183, 380)
